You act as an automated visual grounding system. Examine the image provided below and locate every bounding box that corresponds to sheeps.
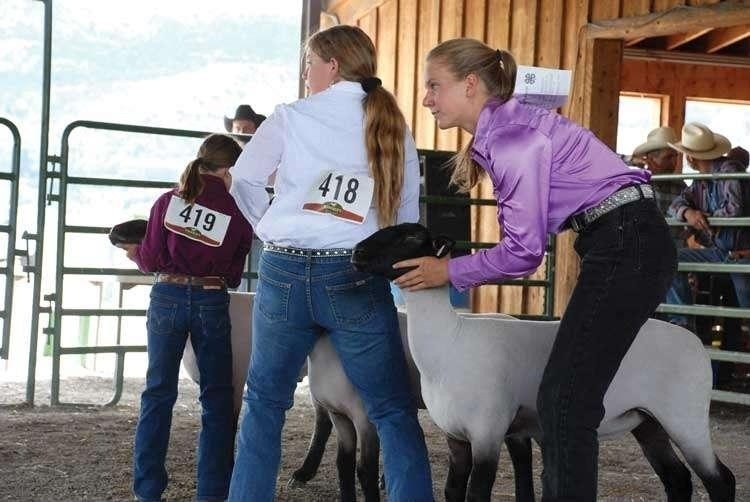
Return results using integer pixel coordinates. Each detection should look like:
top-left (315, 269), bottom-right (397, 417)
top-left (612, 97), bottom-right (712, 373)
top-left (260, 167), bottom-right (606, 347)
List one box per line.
top-left (306, 310), bottom-right (536, 501)
top-left (349, 223), bottom-right (736, 502)
top-left (109, 217), bottom-right (332, 490)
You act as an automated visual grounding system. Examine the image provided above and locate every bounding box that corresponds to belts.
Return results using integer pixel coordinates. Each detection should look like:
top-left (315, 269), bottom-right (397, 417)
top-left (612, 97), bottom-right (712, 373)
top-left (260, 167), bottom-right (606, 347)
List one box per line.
top-left (570, 184), bottom-right (653, 232)
top-left (263, 243), bottom-right (352, 257)
top-left (153, 273), bottom-right (225, 286)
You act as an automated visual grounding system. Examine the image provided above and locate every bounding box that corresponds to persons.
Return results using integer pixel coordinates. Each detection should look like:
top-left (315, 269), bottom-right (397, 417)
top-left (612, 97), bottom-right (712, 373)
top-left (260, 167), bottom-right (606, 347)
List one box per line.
top-left (616, 122), bottom-right (750, 335)
top-left (116, 135), bottom-right (252, 502)
top-left (392, 39), bottom-right (677, 501)
top-left (224, 105), bottom-right (267, 143)
top-left (224, 26), bottom-right (437, 502)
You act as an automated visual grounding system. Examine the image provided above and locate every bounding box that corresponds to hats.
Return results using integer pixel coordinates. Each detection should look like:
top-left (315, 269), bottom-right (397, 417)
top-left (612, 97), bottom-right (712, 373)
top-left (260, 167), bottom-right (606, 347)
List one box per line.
top-left (633, 122), bottom-right (749, 166)
top-left (224, 105), bottom-right (267, 132)
